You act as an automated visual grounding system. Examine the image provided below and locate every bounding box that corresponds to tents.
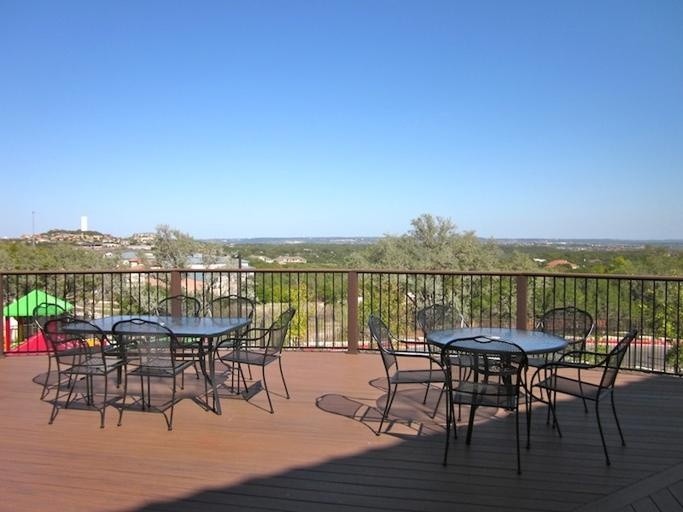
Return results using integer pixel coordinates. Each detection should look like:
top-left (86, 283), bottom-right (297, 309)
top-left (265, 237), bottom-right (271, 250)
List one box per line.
top-left (4, 288), bottom-right (77, 318)
top-left (10, 330), bottom-right (79, 352)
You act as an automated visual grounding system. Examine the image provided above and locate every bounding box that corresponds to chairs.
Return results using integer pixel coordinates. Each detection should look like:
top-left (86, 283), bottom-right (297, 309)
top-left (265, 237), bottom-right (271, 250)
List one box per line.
top-left (44, 317), bottom-right (130, 428)
top-left (527, 329), bottom-right (638, 466)
top-left (112, 318), bottom-right (209, 431)
top-left (140, 295), bottom-right (201, 390)
top-left (441, 337), bottom-right (527, 475)
top-left (182, 295), bottom-right (254, 396)
top-left (212, 308), bottom-right (295, 414)
top-left (33, 303), bottom-right (119, 405)
top-left (511, 307), bottom-right (594, 425)
top-left (416, 304), bottom-right (501, 408)
top-left (368, 316), bottom-right (452, 436)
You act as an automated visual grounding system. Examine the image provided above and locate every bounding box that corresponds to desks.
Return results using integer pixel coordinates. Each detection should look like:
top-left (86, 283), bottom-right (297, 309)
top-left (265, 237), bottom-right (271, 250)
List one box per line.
top-left (58, 315), bottom-right (252, 415)
top-left (426, 327), bottom-right (568, 439)
